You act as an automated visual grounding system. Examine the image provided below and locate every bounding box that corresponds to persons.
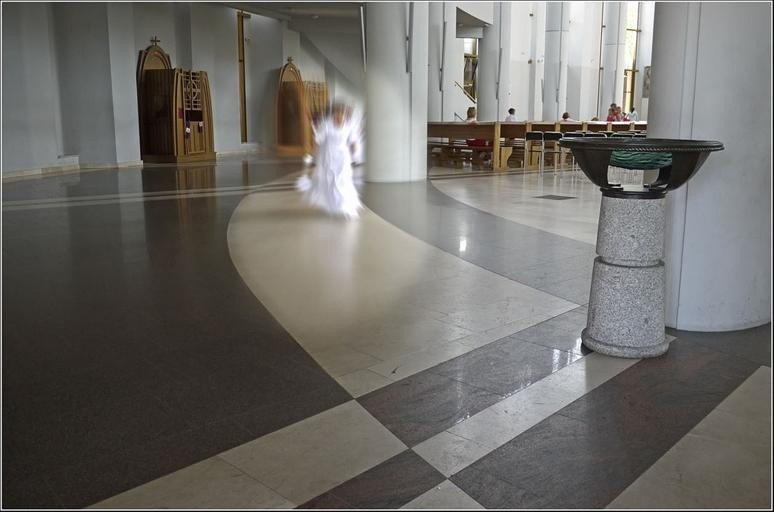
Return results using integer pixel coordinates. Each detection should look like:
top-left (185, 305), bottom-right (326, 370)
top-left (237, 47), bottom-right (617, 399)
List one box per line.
top-left (559, 112), bottom-right (576, 122)
top-left (295, 99), bottom-right (367, 218)
top-left (607, 103), bottom-right (637, 122)
top-left (505, 108), bottom-right (518, 140)
top-left (463, 107), bottom-right (487, 170)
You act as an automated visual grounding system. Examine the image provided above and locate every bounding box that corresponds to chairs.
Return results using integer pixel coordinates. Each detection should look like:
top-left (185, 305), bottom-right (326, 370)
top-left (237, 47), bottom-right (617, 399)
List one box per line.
top-left (526, 130), bottom-right (647, 176)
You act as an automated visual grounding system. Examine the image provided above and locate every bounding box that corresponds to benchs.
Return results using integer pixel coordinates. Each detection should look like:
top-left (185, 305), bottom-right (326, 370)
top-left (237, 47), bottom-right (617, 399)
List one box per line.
top-left (426, 121), bottom-right (647, 169)
